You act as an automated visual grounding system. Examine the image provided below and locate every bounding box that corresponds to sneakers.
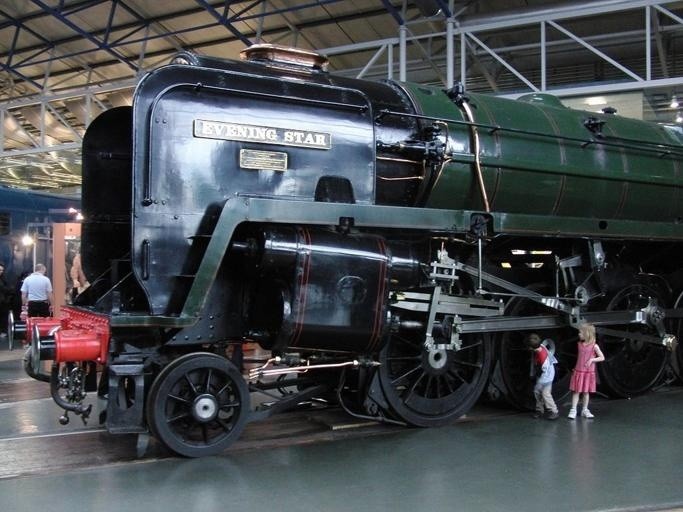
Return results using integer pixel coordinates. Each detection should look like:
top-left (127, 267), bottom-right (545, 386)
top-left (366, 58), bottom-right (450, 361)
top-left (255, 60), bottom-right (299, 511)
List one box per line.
top-left (532, 410), bottom-right (544, 418)
top-left (567, 408), bottom-right (577, 418)
top-left (547, 412), bottom-right (560, 420)
top-left (581, 409), bottom-right (595, 418)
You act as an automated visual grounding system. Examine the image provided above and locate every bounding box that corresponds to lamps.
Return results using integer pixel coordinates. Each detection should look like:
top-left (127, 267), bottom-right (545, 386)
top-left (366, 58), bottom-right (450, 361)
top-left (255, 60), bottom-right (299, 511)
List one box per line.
top-left (673, 85), bottom-right (682, 125)
top-left (670, 38), bottom-right (682, 127)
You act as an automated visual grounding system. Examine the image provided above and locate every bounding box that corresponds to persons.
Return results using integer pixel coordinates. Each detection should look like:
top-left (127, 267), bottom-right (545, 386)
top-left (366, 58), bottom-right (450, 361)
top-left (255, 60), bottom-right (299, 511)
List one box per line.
top-left (523, 334), bottom-right (560, 419)
top-left (568, 324), bottom-right (605, 419)
top-left (70, 254), bottom-right (91, 297)
top-left (20, 263), bottom-right (54, 345)
top-left (0, 264), bottom-right (16, 337)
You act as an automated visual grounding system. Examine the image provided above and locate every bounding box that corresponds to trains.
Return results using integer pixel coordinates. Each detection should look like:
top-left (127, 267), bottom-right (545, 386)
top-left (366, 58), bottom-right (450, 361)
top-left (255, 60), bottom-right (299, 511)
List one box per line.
top-left (0, 185), bottom-right (81, 333)
top-left (6, 42), bottom-right (682, 462)
